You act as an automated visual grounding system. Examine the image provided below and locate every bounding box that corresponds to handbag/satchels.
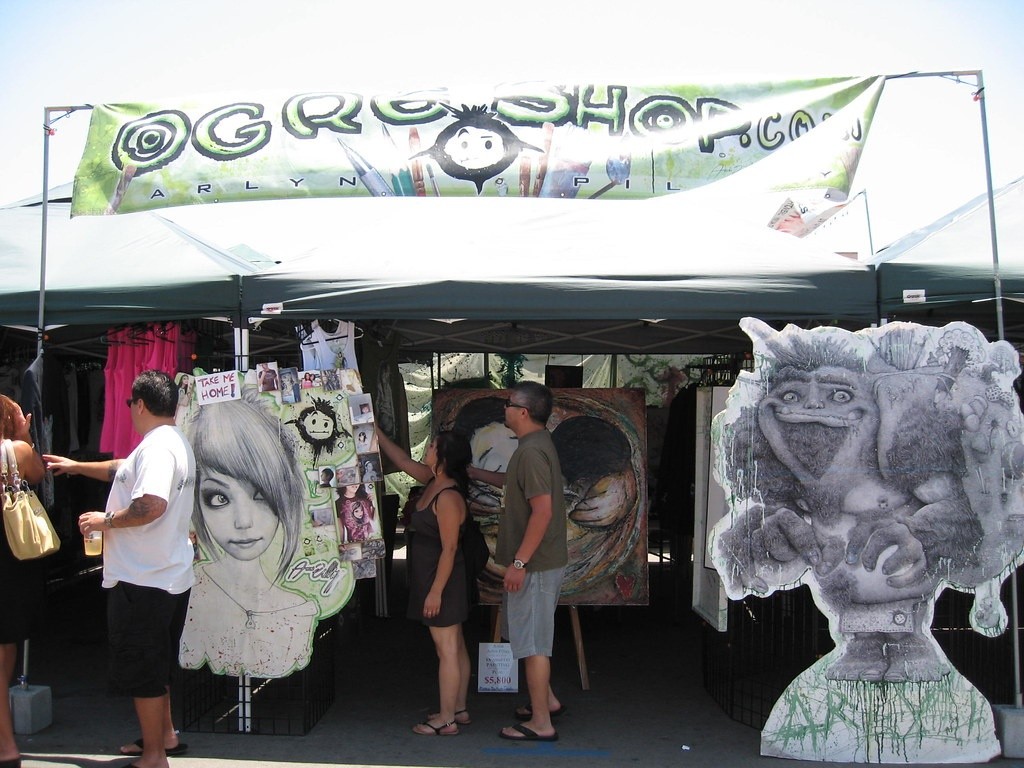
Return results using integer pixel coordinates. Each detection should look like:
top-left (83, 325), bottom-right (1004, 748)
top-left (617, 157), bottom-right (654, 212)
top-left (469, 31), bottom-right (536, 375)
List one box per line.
top-left (0, 438), bottom-right (61, 560)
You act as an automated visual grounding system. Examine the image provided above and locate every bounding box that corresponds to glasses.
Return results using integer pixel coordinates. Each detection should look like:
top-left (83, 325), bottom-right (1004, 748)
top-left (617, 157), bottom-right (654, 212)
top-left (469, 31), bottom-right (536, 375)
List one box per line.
top-left (506, 396), bottom-right (529, 412)
top-left (126, 398), bottom-right (140, 408)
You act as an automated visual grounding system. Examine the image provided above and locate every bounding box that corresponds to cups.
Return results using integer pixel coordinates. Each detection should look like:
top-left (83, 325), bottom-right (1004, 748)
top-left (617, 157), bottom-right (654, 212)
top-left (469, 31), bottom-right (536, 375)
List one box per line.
top-left (80, 514), bottom-right (103, 556)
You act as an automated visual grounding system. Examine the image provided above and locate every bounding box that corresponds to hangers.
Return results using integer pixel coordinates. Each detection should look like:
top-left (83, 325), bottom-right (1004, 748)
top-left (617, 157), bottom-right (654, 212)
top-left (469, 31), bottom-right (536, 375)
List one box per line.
top-left (295, 314), bottom-right (363, 346)
top-left (1, 319), bottom-right (223, 375)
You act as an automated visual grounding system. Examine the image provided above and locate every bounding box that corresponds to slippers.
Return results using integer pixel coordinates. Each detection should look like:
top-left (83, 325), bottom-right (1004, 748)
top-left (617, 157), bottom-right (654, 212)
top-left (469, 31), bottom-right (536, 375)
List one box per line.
top-left (514, 703), bottom-right (567, 721)
top-left (121, 738), bottom-right (188, 754)
top-left (500, 722), bottom-right (559, 741)
top-left (0, 755), bottom-right (22, 768)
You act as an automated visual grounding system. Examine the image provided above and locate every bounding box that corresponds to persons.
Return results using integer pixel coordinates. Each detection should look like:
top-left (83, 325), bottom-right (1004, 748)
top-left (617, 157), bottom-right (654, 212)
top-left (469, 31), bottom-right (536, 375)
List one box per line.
top-left (0, 393), bottom-right (45, 768)
top-left (323, 374), bottom-right (342, 391)
top-left (335, 484), bottom-right (376, 544)
top-left (304, 536), bottom-right (329, 556)
top-left (279, 369), bottom-right (301, 404)
top-left (358, 432), bottom-right (368, 445)
top-left (466, 381), bottom-right (568, 740)
top-left (258, 364), bottom-right (278, 392)
top-left (339, 545), bottom-right (357, 560)
top-left (320, 468), bottom-right (334, 487)
top-left (302, 372), bottom-right (322, 388)
top-left (363, 461), bottom-right (380, 482)
top-left (362, 540), bottom-right (385, 559)
top-left (176, 375), bottom-right (192, 405)
top-left (42, 369), bottom-right (196, 768)
top-left (374, 418), bottom-right (479, 735)
top-left (360, 404), bottom-right (370, 414)
top-left (354, 560), bottom-right (375, 577)
top-left (337, 469), bottom-right (353, 483)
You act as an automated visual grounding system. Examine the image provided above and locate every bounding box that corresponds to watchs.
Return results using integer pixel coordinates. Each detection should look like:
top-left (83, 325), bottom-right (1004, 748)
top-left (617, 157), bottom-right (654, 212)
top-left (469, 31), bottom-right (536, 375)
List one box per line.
top-left (513, 558), bottom-right (528, 569)
top-left (105, 511), bottom-right (114, 528)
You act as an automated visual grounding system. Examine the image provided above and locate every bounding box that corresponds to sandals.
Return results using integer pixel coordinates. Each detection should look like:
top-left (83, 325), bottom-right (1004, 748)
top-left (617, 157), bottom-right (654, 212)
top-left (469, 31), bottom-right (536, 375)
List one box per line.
top-left (427, 709), bottom-right (471, 724)
top-left (413, 720), bottom-right (459, 735)
